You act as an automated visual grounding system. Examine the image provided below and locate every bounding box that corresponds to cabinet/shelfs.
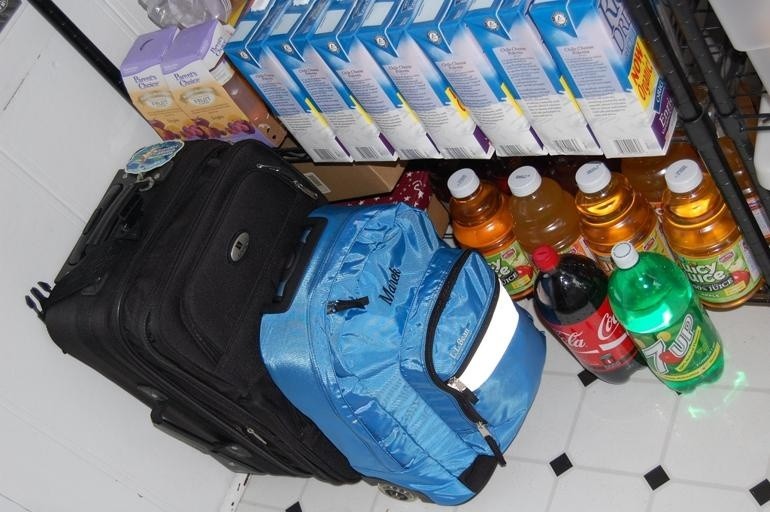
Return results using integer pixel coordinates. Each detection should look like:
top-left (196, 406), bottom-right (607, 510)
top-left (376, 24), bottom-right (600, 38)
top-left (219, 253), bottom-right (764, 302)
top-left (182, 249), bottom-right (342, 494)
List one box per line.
top-left (23, 0), bottom-right (768, 307)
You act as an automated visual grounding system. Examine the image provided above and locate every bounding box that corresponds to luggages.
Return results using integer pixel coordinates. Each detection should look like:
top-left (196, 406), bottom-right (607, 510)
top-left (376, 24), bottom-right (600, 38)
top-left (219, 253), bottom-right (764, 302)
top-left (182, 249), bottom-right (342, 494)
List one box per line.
top-left (258, 202), bottom-right (544, 505)
top-left (23, 138), bottom-right (362, 487)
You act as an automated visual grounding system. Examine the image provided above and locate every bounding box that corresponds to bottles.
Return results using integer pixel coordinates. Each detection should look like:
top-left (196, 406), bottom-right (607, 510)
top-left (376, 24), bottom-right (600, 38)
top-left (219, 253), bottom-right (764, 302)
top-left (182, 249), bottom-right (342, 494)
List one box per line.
top-left (447, 168), bottom-right (539, 301)
top-left (658, 166), bottom-right (763, 312)
top-left (575, 161), bottom-right (677, 276)
top-left (618, 84), bottom-right (766, 214)
top-left (606, 242), bottom-right (727, 394)
top-left (530, 245), bottom-right (646, 384)
top-left (506, 166), bottom-right (599, 276)
